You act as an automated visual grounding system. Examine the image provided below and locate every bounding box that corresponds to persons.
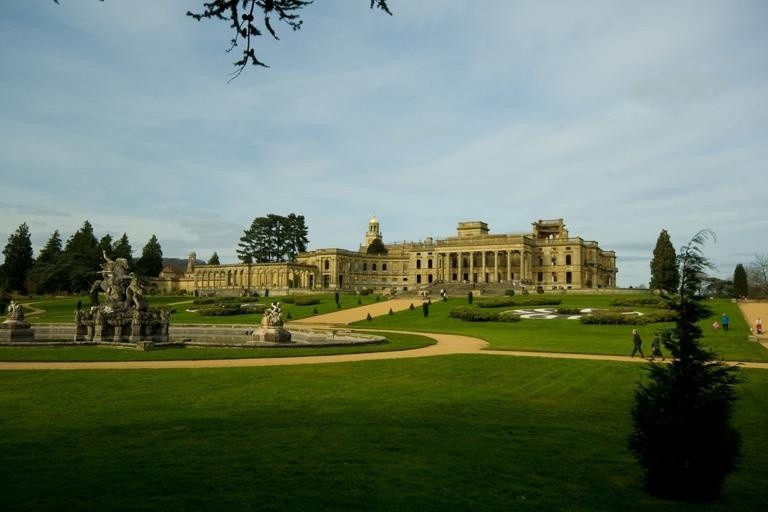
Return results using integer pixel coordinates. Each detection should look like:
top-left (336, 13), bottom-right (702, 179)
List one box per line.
top-left (648, 331), bottom-right (666, 361)
top-left (443, 288), bottom-right (448, 300)
top-left (6, 299), bottom-right (26, 319)
top-left (264, 300), bottom-right (283, 325)
top-left (631, 328), bottom-right (645, 358)
top-left (472, 282), bottom-right (476, 288)
top-left (69, 246), bottom-right (175, 344)
top-left (721, 312), bottom-right (731, 330)
top-left (439, 287), bottom-right (444, 297)
top-left (712, 322), bottom-right (720, 329)
top-left (755, 317), bottom-right (763, 334)
